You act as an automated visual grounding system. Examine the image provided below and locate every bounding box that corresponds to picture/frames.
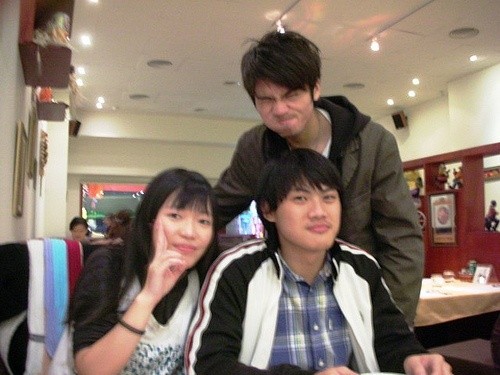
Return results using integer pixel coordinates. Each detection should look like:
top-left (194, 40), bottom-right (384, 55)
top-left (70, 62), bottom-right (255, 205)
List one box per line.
top-left (12, 120), bottom-right (29, 217)
top-left (427, 190), bottom-right (460, 249)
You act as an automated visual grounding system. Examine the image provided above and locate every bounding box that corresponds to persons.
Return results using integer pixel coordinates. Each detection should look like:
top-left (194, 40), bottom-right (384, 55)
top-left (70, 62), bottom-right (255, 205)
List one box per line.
top-left (486, 201), bottom-right (500, 231)
top-left (215, 29), bottom-right (424, 334)
top-left (182, 147), bottom-right (452, 375)
top-left (69, 167), bottom-right (219, 375)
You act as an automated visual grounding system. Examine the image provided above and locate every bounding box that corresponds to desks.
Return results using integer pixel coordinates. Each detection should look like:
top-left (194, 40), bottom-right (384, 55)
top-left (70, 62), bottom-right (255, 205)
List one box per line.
top-left (413, 277), bottom-right (500, 375)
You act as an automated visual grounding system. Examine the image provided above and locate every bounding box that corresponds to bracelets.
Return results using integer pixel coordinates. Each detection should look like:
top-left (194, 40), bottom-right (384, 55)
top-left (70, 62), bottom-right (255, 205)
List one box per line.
top-left (119, 316), bottom-right (145, 335)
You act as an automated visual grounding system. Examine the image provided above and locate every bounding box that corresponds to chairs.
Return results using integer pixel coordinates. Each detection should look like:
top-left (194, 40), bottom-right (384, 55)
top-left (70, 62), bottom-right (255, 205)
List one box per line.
top-left (0, 241), bottom-right (111, 375)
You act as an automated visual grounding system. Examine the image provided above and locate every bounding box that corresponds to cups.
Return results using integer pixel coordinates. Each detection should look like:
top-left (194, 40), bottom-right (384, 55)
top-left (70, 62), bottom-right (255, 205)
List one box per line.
top-left (442, 271), bottom-right (454, 289)
top-left (431, 273), bottom-right (442, 291)
